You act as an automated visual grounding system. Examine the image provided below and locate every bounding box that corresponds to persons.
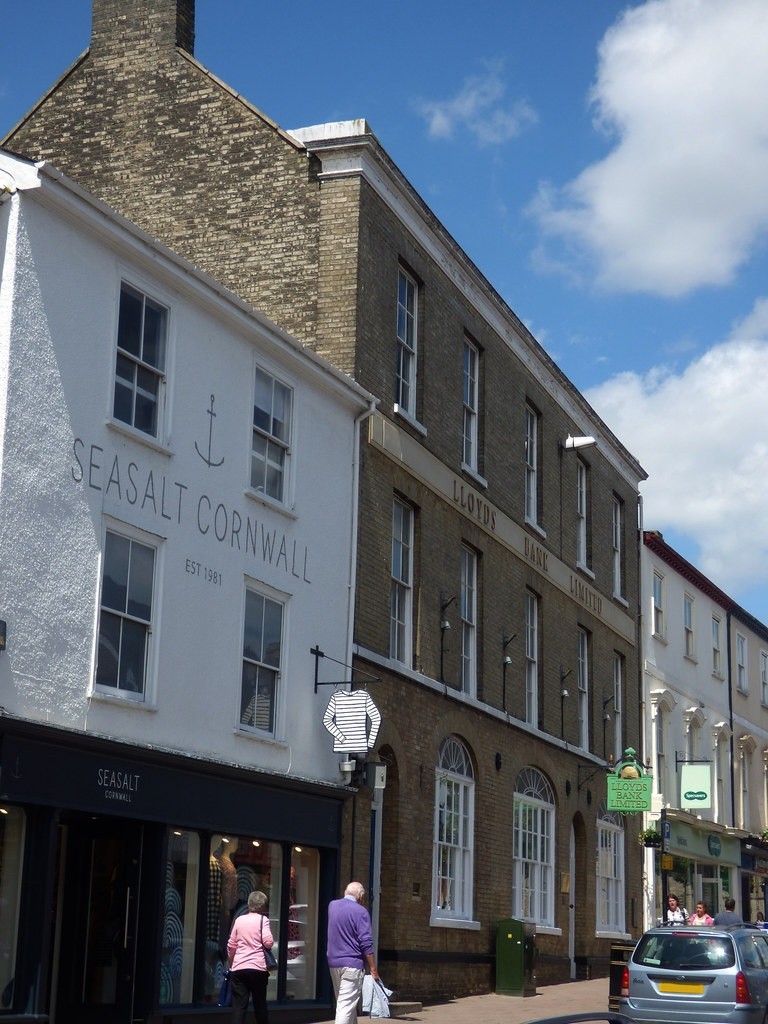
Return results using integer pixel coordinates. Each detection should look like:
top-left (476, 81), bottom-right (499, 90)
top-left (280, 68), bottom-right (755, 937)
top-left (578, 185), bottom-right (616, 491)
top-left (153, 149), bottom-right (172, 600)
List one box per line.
top-left (207, 835), bottom-right (237, 950)
top-left (327, 882), bottom-right (379, 1024)
top-left (227, 891), bottom-right (273, 1024)
top-left (661, 893), bottom-right (744, 927)
top-left (756, 912), bottom-right (766, 923)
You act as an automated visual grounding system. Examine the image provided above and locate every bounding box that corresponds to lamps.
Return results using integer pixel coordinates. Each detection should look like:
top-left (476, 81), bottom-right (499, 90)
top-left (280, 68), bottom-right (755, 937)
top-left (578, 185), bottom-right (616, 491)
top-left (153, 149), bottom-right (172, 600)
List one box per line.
top-left (560, 433), bottom-right (598, 452)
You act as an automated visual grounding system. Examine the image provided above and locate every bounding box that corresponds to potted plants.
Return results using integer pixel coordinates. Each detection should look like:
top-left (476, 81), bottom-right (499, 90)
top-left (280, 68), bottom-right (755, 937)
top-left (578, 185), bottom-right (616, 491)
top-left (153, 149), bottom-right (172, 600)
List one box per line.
top-left (638, 822), bottom-right (663, 848)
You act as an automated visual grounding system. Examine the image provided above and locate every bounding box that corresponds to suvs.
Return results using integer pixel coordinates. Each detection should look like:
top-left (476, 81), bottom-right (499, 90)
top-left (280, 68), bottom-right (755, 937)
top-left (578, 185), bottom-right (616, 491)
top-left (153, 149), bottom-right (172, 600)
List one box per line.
top-left (619, 921), bottom-right (768, 1024)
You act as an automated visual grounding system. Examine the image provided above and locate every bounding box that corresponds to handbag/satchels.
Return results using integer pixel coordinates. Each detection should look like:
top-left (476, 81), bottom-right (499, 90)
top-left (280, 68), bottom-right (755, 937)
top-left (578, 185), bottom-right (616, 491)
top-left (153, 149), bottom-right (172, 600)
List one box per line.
top-left (215, 967), bottom-right (233, 1014)
top-left (263, 947), bottom-right (278, 972)
